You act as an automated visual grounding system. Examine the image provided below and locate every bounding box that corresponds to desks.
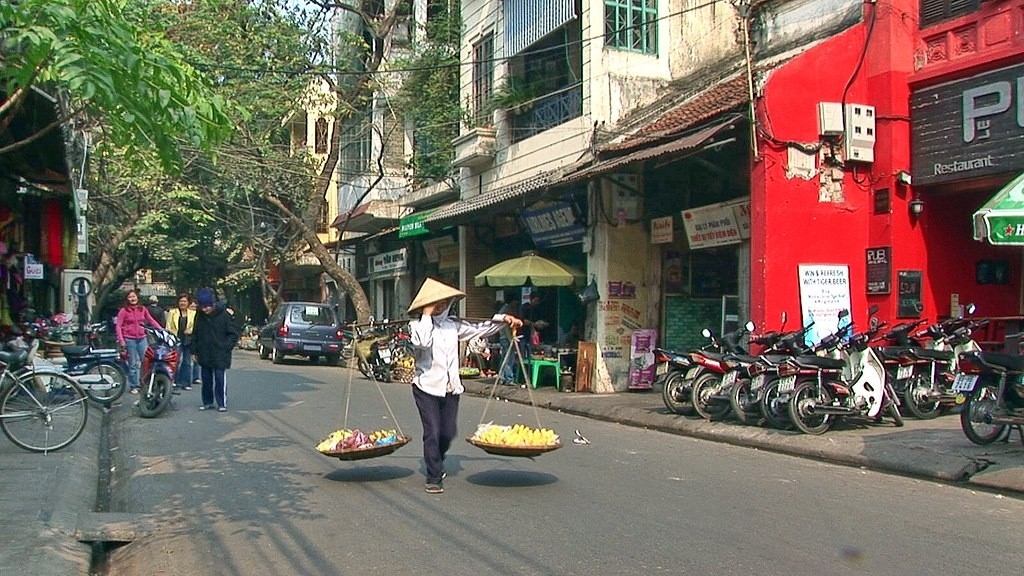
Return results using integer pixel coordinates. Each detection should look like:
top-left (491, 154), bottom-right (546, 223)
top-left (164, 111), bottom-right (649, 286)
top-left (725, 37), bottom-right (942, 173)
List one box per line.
top-left (516, 358), bottom-right (560, 389)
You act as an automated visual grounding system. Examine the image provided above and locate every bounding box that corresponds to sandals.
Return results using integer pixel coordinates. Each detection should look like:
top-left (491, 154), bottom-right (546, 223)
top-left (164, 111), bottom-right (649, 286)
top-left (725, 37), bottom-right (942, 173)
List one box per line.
top-left (425, 471), bottom-right (447, 493)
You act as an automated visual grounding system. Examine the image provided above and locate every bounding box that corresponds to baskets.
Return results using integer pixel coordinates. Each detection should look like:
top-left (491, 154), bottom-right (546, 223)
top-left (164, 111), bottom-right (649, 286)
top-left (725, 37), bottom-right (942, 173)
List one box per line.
top-left (393, 366), bottom-right (415, 383)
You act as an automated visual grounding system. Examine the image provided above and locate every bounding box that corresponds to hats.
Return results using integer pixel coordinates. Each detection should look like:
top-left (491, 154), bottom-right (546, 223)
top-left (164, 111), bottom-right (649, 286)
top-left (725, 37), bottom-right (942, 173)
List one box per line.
top-left (197, 288), bottom-right (216, 307)
top-left (406, 277), bottom-right (468, 313)
top-left (149, 295), bottom-right (158, 302)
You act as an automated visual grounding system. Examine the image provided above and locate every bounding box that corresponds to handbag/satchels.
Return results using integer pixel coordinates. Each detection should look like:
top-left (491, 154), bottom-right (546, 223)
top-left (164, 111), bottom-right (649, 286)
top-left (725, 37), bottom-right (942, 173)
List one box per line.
top-left (530, 322), bottom-right (539, 346)
top-left (575, 274), bottom-right (600, 305)
top-left (184, 335), bottom-right (192, 346)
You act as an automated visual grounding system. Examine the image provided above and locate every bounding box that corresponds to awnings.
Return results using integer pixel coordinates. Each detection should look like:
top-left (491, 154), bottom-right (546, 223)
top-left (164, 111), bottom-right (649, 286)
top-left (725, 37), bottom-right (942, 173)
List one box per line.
top-left (971, 170), bottom-right (1024, 246)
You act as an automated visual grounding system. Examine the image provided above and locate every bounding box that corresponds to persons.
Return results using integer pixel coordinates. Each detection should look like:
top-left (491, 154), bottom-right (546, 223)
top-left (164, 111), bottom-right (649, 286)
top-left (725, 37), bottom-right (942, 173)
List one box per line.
top-left (408, 276), bottom-right (524, 494)
top-left (522, 291), bottom-right (549, 360)
top-left (189, 289), bottom-right (240, 411)
top-left (497, 293), bottom-right (530, 386)
top-left (112, 291), bottom-right (202, 396)
top-left (559, 321), bottom-right (581, 371)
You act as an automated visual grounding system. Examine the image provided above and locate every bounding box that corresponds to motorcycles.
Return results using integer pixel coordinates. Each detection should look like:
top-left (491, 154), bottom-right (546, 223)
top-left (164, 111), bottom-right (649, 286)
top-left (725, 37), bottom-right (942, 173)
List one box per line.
top-left (340, 316), bottom-right (414, 380)
top-left (135, 323), bottom-right (182, 418)
top-left (0, 317), bottom-right (126, 409)
top-left (652, 302), bottom-right (991, 436)
top-left (951, 334), bottom-right (1024, 445)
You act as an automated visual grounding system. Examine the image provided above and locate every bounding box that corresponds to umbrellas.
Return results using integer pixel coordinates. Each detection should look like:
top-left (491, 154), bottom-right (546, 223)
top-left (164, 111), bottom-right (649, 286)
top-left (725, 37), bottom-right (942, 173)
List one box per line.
top-left (472, 254), bottom-right (588, 357)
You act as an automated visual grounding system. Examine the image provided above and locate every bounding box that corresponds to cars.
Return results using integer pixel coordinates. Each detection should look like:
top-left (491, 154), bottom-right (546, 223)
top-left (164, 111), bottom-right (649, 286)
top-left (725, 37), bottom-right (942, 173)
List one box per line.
top-left (258, 301), bottom-right (342, 366)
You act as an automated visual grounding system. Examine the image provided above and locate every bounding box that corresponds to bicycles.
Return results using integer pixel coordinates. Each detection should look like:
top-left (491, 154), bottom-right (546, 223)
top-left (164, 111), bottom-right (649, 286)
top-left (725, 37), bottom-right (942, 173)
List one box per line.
top-left (0, 350), bottom-right (88, 457)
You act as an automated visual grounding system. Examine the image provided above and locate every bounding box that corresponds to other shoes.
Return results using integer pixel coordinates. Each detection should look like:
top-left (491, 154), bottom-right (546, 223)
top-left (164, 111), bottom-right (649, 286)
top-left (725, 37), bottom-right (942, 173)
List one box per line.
top-left (479, 371), bottom-right (486, 378)
top-left (486, 369), bottom-right (496, 374)
top-left (500, 381), bottom-right (517, 386)
top-left (193, 378), bottom-right (202, 384)
top-left (131, 388), bottom-right (138, 394)
top-left (218, 407), bottom-right (227, 411)
top-left (198, 404), bottom-right (215, 410)
top-left (184, 386), bottom-right (193, 391)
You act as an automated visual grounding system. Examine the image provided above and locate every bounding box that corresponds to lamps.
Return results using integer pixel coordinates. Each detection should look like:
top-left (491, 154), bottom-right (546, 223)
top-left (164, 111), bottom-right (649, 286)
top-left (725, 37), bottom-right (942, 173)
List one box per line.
top-left (900, 170), bottom-right (911, 186)
top-left (910, 192), bottom-right (924, 218)
